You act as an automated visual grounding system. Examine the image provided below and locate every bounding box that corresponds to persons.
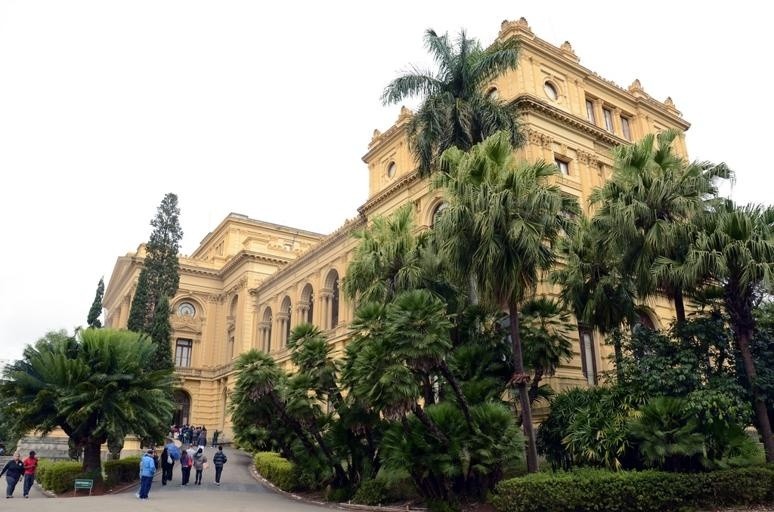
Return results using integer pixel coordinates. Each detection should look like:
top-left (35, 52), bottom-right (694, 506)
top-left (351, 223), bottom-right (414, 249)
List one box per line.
top-left (135, 424), bottom-right (227, 499)
top-left (22, 450), bottom-right (38, 498)
top-left (0, 451), bottom-right (24, 498)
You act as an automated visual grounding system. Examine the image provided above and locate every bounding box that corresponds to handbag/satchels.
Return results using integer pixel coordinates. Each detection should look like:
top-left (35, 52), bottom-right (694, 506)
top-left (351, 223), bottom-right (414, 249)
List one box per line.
top-left (188, 459), bottom-right (192, 467)
top-left (167, 456), bottom-right (173, 464)
